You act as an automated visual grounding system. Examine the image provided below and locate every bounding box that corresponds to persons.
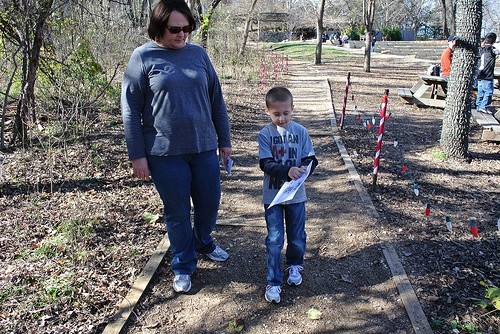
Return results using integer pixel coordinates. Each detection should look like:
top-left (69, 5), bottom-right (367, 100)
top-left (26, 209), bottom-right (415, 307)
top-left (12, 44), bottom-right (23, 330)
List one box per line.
top-left (476, 33), bottom-right (496, 114)
top-left (371, 35), bottom-right (376, 52)
top-left (441, 35), bottom-right (456, 81)
top-left (336, 32), bottom-right (342, 45)
top-left (343, 33), bottom-right (348, 43)
top-left (322, 31), bottom-right (329, 42)
top-left (121, 0), bottom-right (231, 295)
top-left (259, 86), bottom-right (320, 304)
top-left (329, 34), bottom-right (334, 44)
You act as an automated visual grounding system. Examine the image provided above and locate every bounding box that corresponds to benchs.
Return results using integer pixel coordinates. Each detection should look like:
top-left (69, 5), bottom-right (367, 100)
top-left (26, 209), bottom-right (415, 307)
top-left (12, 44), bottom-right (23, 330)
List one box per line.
top-left (471, 108), bottom-right (500, 141)
top-left (397, 88), bottom-right (412, 103)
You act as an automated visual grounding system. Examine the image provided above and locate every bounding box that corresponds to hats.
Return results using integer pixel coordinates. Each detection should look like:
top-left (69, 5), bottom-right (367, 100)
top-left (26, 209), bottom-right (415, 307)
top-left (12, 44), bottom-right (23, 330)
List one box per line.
top-left (448, 36), bottom-right (456, 41)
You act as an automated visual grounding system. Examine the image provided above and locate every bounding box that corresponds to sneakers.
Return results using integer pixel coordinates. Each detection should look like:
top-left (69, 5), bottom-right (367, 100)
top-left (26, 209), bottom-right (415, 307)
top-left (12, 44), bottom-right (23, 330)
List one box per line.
top-left (205, 245), bottom-right (228, 261)
top-left (173, 274), bottom-right (191, 292)
top-left (265, 285), bottom-right (281, 303)
top-left (479, 107), bottom-right (492, 114)
top-left (286, 265), bottom-right (303, 286)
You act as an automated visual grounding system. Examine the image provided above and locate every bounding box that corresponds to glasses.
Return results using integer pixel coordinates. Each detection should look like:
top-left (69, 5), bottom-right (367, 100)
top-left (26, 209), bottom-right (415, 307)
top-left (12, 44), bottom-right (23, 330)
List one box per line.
top-left (165, 24), bottom-right (191, 34)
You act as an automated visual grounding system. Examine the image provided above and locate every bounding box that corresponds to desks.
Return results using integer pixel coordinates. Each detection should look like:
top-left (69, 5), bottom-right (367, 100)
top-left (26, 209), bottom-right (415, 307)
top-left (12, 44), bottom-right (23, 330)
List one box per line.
top-left (409, 74), bottom-right (447, 108)
